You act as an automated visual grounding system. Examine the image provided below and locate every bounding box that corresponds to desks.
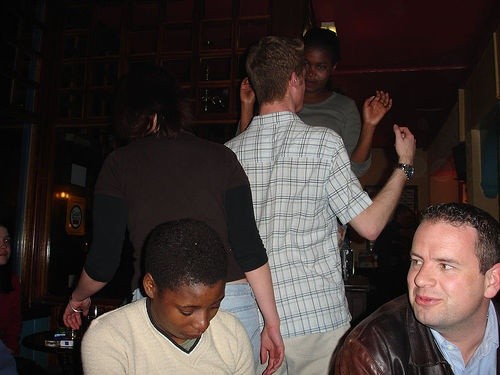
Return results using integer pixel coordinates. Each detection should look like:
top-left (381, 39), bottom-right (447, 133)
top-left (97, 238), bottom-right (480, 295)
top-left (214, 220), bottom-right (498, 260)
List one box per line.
top-left (21, 331), bottom-right (81, 375)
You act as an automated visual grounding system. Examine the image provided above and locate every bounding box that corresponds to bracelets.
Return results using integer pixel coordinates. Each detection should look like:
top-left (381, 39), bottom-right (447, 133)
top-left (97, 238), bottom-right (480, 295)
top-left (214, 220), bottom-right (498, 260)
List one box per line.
top-left (68, 294), bottom-right (93, 314)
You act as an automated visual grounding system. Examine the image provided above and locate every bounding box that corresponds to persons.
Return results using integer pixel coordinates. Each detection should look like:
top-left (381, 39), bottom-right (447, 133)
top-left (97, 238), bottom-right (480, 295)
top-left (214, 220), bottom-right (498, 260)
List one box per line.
top-left (0, 226), bottom-right (24, 375)
top-left (79, 218), bottom-right (254, 375)
top-left (222, 34), bottom-right (418, 375)
top-left (62, 81), bottom-right (286, 375)
top-left (330, 200), bottom-right (500, 375)
top-left (235, 27), bottom-right (393, 180)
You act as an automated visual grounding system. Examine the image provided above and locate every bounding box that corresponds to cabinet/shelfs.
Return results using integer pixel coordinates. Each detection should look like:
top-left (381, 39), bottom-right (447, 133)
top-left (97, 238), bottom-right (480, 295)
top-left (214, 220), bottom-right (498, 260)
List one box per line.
top-left (39, 0), bottom-right (271, 303)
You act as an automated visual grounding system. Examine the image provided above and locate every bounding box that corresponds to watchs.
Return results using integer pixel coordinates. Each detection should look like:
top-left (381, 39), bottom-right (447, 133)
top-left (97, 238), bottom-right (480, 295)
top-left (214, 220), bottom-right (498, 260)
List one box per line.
top-left (396, 160), bottom-right (418, 183)
